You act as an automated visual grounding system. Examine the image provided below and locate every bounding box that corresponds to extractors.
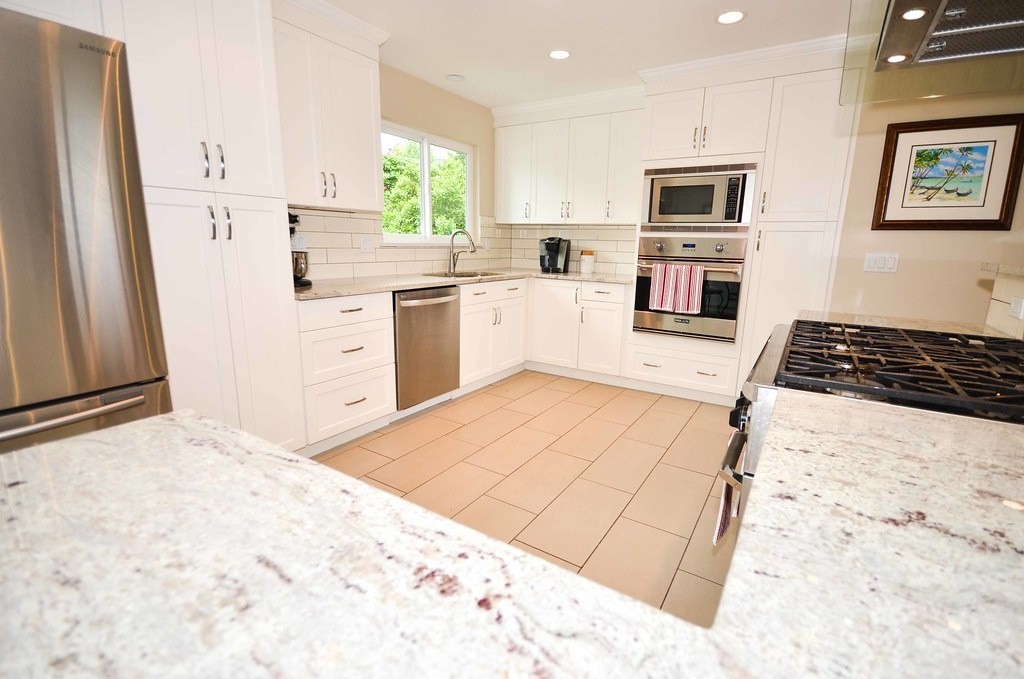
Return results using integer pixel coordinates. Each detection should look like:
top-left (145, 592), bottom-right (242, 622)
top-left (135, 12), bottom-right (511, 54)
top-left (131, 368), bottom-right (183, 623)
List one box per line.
top-left (876, 3), bottom-right (1024, 76)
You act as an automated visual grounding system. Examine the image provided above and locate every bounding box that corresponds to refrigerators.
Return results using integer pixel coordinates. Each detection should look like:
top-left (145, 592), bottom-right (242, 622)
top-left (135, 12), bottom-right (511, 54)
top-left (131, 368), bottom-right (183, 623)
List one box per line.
top-left (0, 7), bottom-right (173, 456)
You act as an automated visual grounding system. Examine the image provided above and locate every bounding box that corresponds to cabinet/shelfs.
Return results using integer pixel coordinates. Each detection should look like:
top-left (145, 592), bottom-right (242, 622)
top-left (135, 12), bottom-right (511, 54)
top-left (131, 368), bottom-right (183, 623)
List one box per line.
top-left (105, 0), bottom-right (289, 198)
top-left (272, 0), bottom-right (387, 212)
top-left (496, 109), bottom-right (645, 223)
top-left (626, 329), bottom-right (748, 401)
top-left (297, 292), bottom-right (400, 455)
top-left (640, 75), bottom-right (778, 161)
top-left (143, 204), bottom-right (308, 453)
top-left (455, 280), bottom-right (529, 390)
top-left (529, 278), bottom-right (631, 374)
top-left (736, 230), bottom-right (838, 401)
top-left (754, 69), bottom-right (866, 221)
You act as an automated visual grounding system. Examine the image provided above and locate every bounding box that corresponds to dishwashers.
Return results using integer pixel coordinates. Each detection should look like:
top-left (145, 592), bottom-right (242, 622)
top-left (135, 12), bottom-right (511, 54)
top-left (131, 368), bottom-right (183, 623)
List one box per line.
top-left (393, 286), bottom-right (460, 408)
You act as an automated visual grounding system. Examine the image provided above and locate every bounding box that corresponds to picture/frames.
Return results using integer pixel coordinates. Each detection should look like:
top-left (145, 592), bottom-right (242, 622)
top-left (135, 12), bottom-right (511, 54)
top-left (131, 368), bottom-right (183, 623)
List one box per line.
top-left (872, 113), bottom-right (1024, 230)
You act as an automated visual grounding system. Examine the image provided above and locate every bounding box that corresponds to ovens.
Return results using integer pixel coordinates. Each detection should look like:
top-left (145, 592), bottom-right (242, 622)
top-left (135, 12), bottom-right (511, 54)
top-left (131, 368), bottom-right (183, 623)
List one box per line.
top-left (632, 236), bottom-right (748, 344)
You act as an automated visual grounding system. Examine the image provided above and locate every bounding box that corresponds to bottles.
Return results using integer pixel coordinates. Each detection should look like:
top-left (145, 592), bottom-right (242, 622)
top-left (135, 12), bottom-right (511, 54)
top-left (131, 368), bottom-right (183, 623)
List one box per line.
top-left (578, 251), bottom-right (594, 273)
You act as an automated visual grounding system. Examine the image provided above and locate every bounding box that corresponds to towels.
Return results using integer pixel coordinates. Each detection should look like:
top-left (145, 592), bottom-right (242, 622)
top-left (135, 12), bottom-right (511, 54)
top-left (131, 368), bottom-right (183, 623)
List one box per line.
top-left (712, 429), bottom-right (749, 547)
top-left (649, 263), bottom-right (678, 312)
top-left (674, 265), bottom-right (704, 314)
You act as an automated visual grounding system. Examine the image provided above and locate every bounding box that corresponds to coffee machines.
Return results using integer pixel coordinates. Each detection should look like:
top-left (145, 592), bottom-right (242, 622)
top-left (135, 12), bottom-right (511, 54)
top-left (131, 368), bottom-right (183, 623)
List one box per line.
top-left (288, 213), bottom-right (313, 288)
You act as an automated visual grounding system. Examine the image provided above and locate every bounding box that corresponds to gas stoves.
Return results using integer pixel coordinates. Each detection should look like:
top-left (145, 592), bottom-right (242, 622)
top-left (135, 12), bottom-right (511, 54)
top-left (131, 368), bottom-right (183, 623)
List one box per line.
top-left (771, 318), bottom-right (1024, 424)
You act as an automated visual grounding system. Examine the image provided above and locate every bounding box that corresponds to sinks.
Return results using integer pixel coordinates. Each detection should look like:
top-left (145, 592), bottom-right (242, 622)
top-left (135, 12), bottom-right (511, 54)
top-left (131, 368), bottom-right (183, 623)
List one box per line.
top-left (437, 274), bottom-right (498, 277)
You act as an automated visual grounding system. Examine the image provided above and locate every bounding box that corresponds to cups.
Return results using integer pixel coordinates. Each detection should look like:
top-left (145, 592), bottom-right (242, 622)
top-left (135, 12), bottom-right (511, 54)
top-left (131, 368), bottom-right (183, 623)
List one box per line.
top-left (291, 250), bottom-right (308, 281)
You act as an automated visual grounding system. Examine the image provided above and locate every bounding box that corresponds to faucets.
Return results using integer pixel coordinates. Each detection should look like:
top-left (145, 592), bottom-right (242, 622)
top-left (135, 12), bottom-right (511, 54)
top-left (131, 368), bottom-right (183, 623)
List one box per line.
top-left (448, 229), bottom-right (477, 274)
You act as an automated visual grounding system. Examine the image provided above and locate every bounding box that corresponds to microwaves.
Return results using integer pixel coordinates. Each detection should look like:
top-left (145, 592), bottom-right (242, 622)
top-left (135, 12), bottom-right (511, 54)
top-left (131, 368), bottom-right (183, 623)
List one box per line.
top-left (650, 175), bottom-right (745, 224)
